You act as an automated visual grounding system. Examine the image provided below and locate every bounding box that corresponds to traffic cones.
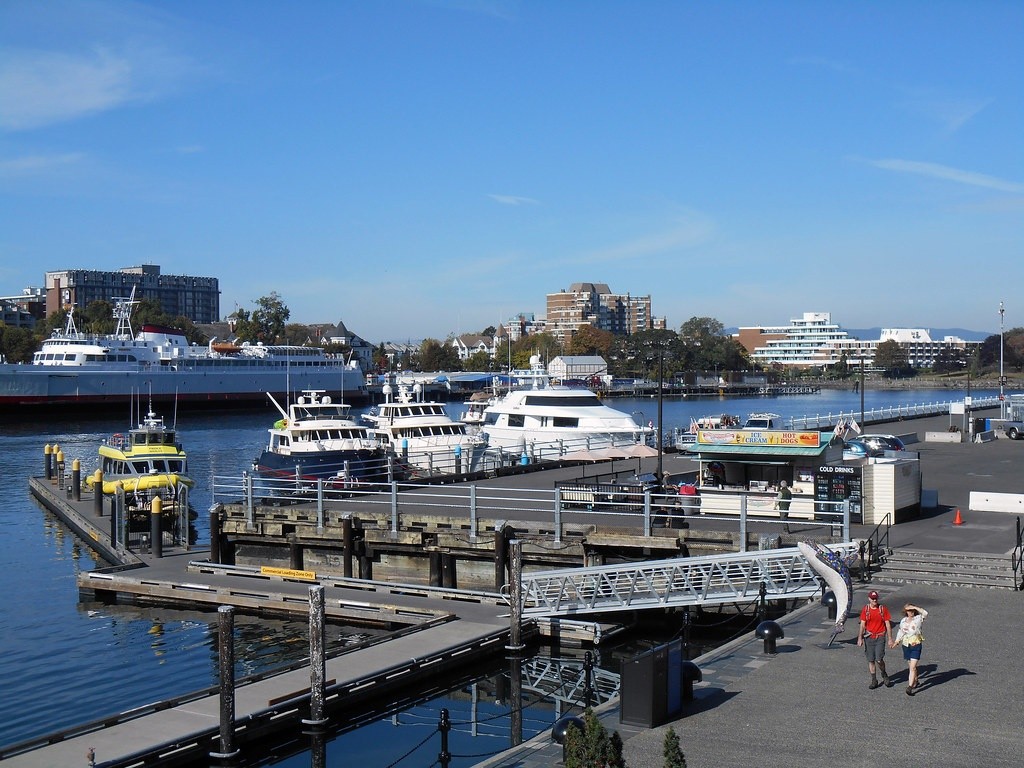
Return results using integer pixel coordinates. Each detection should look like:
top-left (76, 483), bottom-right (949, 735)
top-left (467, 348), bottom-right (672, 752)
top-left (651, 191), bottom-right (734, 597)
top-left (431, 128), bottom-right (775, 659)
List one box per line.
top-left (952, 510), bottom-right (963, 525)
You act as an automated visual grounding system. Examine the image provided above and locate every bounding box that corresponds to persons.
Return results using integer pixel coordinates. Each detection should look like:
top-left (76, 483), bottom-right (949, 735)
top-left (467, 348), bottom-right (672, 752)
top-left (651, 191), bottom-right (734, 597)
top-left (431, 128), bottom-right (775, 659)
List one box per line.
top-left (663, 471), bottom-right (679, 502)
top-left (692, 475), bottom-right (700, 486)
top-left (857, 591), bottom-right (894, 689)
top-left (773, 480), bottom-right (791, 534)
top-left (892, 603), bottom-right (928, 694)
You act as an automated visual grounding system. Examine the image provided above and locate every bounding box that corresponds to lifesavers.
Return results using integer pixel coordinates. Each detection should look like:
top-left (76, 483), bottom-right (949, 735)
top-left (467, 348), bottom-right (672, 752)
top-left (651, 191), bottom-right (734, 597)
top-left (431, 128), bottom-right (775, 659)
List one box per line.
top-left (113, 433), bottom-right (124, 446)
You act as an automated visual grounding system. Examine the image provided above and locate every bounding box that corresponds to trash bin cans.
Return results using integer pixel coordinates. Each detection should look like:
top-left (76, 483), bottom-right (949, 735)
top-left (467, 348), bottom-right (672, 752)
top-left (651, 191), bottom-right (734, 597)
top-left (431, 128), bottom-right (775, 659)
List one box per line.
top-left (679, 486), bottom-right (696, 515)
top-left (975, 417), bottom-right (991, 438)
top-left (619, 636), bottom-right (686, 730)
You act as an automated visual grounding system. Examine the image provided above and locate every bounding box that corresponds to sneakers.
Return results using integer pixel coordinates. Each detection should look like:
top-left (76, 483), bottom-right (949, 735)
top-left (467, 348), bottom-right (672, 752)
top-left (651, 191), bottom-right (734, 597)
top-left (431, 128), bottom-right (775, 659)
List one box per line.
top-left (783, 530), bottom-right (790, 535)
top-left (906, 686), bottom-right (912, 696)
top-left (881, 672), bottom-right (890, 687)
top-left (869, 673), bottom-right (878, 689)
top-left (913, 680), bottom-right (920, 688)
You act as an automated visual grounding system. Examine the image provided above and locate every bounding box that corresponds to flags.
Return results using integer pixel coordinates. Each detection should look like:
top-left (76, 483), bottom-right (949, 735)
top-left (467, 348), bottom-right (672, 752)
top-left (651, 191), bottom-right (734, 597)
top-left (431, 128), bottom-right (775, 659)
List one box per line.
top-left (689, 415), bottom-right (712, 434)
top-left (834, 417), bottom-right (861, 437)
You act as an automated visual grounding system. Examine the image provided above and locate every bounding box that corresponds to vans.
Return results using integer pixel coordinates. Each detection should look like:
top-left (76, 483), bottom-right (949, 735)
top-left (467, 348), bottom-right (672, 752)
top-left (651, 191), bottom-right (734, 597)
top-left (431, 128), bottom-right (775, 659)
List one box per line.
top-left (741, 415), bottom-right (785, 431)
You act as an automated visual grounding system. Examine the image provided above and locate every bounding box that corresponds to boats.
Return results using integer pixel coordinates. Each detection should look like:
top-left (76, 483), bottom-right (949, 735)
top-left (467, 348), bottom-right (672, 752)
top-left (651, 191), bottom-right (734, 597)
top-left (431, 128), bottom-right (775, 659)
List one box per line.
top-left (0, 280), bottom-right (372, 423)
top-left (82, 379), bottom-right (198, 526)
top-left (252, 338), bottom-right (384, 500)
top-left (473, 346), bottom-right (667, 466)
top-left (213, 342), bottom-right (241, 353)
top-left (359, 364), bottom-right (488, 480)
top-left (457, 345), bottom-right (570, 429)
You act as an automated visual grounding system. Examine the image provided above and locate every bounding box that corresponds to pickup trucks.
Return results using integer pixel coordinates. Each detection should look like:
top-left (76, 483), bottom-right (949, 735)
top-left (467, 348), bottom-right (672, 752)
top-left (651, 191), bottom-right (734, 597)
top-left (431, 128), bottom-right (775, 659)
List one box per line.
top-left (561, 379), bottom-right (586, 386)
top-left (674, 415), bottom-right (744, 454)
top-left (1003, 419), bottom-right (1024, 440)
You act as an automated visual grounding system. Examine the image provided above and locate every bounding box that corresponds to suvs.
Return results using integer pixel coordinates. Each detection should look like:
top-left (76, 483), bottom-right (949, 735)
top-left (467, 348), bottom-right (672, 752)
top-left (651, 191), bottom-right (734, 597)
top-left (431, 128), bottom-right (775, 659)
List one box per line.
top-left (847, 434), bottom-right (906, 458)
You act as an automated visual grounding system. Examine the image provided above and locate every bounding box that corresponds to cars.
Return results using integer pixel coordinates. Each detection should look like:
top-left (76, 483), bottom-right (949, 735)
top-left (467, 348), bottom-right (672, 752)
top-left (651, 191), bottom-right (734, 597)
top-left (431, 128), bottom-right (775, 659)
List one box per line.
top-left (843, 442), bottom-right (876, 460)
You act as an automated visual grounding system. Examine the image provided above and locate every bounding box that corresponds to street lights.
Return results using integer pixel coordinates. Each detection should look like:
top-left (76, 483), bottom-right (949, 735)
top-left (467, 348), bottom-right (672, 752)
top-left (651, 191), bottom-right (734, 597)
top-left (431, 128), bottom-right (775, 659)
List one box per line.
top-left (858, 355), bottom-right (867, 433)
top-left (997, 302), bottom-right (1006, 419)
top-left (714, 364), bottom-right (719, 386)
top-left (751, 361), bottom-right (756, 385)
top-left (646, 351), bottom-right (663, 491)
top-left (964, 344), bottom-right (973, 432)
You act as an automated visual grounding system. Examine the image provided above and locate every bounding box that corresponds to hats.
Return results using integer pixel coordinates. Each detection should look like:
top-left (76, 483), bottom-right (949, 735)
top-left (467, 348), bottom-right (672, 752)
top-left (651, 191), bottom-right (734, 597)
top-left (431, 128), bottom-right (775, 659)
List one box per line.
top-left (868, 591), bottom-right (879, 599)
top-left (781, 481), bottom-right (787, 488)
